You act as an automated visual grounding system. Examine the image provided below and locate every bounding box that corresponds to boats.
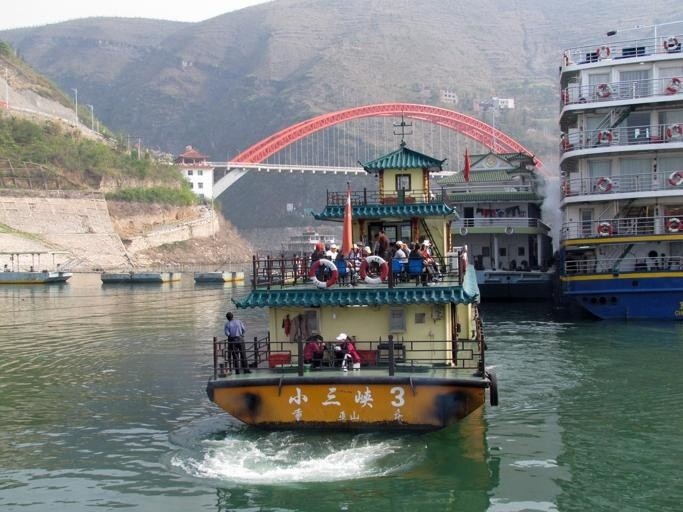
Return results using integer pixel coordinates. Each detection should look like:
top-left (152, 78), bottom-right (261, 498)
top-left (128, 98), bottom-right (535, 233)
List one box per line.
top-left (193, 271), bottom-right (244, 282)
top-left (99, 270), bottom-right (182, 283)
top-left (0, 250), bottom-right (72, 284)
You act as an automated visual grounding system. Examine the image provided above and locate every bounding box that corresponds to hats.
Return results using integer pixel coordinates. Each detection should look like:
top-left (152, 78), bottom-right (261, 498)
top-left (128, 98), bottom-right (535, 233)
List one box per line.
top-left (330, 245), bottom-right (336, 249)
top-left (336, 333), bottom-right (346, 341)
top-left (364, 246), bottom-right (370, 253)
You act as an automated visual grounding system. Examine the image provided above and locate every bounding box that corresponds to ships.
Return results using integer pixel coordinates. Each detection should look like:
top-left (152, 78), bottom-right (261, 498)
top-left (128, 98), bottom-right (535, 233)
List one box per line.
top-left (554, 22), bottom-right (682, 323)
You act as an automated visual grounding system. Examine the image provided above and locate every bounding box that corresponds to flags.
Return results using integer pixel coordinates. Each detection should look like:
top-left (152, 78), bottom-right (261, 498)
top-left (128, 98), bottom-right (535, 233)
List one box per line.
top-left (342, 191), bottom-right (352, 256)
top-left (464, 147), bottom-right (470, 182)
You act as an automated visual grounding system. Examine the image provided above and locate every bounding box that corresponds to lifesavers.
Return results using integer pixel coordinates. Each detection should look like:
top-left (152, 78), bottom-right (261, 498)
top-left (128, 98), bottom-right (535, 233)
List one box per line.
top-left (597, 177), bottom-right (613, 192)
top-left (597, 222), bottom-right (613, 237)
top-left (459, 227), bottom-right (468, 236)
top-left (360, 255), bottom-right (389, 284)
top-left (596, 83), bottom-right (613, 97)
top-left (667, 123), bottom-right (683, 139)
top-left (309, 259), bottom-right (339, 287)
top-left (504, 226), bottom-right (513, 235)
top-left (597, 45), bottom-right (610, 59)
top-left (666, 218), bottom-right (681, 233)
top-left (665, 77), bottom-right (681, 93)
top-left (464, 252), bottom-right (467, 273)
top-left (669, 172), bottom-right (683, 185)
top-left (663, 36), bottom-right (679, 51)
top-left (598, 130), bottom-right (612, 144)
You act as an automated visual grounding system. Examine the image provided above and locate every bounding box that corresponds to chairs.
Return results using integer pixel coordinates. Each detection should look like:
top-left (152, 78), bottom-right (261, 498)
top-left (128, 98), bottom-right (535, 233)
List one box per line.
top-left (311, 258), bottom-right (355, 288)
top-left (391, 258), bottom-right (427, 286)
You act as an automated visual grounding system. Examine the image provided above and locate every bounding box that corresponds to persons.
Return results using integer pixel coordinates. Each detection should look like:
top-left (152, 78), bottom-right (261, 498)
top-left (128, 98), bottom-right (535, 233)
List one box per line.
top-left (225, 312), bottom-right (252, 374)
top-left (334, 333), bottom-right (361, 372)
top-left (4, 265), bottom-right (7, 272)
top-left (31, 266), bottom-right (33, 272)
top-left (312, 230), bottom-right (445, 288)
top-left (304, 336), bottom-right (325, 370)
top-left (57, 264), bottom-right (60, 272)
